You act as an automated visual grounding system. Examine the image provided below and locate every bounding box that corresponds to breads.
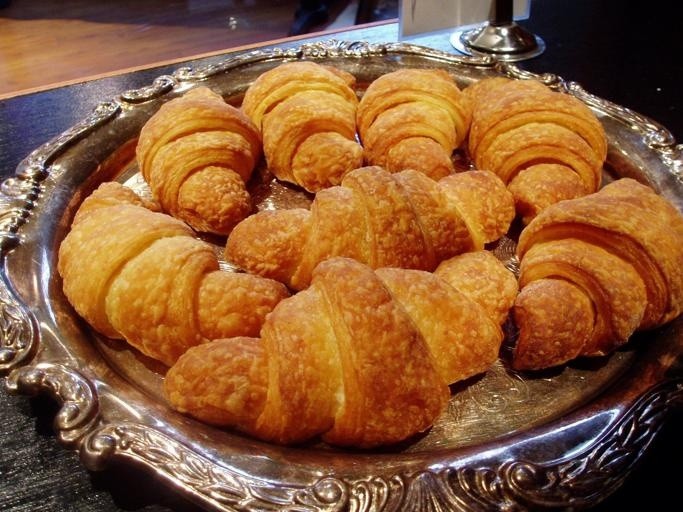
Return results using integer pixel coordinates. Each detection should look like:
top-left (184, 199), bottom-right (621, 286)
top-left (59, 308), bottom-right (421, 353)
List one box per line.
top-left (57, 61), bottom-right (683, 449)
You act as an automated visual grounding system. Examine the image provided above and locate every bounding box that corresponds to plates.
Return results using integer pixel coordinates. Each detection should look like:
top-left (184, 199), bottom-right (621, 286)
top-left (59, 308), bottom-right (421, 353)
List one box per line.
top-left (0, 38), bottom-right (682, 512)
